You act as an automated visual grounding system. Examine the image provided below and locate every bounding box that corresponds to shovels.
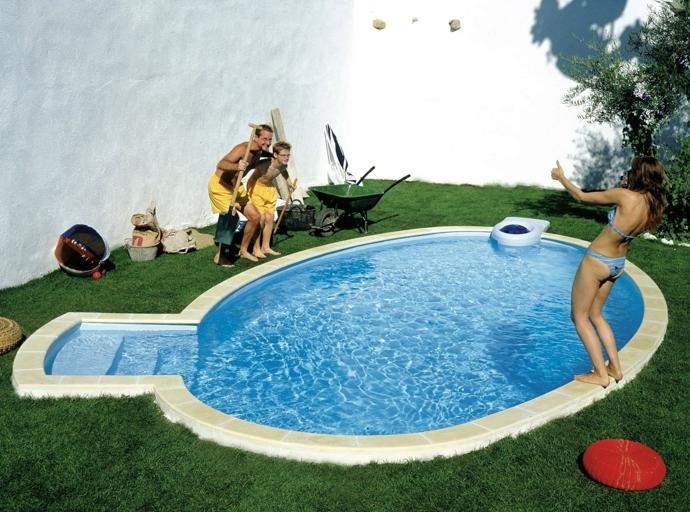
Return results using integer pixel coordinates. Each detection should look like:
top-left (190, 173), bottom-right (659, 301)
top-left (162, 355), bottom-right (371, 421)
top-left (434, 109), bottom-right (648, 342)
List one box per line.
top-left (215, 123), bottom-right (263, 245)
top-left (271, 178), bottom-right (298, 247)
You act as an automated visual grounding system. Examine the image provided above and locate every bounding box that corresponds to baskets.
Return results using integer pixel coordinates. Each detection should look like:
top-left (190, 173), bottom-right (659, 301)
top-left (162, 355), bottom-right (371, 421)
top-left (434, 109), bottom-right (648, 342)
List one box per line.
top-left (276, 199), bottom-right (316, 230)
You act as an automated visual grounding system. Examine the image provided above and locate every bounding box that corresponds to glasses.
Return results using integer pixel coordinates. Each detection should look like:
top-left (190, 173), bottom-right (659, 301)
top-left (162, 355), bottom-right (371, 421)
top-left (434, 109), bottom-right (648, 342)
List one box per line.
top-left (278, 153), bottom-right (291, 157)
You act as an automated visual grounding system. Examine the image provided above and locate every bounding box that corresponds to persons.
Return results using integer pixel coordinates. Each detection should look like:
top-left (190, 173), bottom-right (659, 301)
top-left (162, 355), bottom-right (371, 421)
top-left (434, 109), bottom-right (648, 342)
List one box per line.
top-left (248, 142), bottom-right (297, 258)
top-left (206, 124), bottom-right (274, 268)
top-left (550, 156), bottom-right (665, 385)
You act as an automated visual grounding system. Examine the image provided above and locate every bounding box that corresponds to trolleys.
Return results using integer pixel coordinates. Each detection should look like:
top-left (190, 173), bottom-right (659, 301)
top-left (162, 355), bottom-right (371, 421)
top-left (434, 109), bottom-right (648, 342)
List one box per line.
top-left (307, 166), bottom-right (410, 238)
